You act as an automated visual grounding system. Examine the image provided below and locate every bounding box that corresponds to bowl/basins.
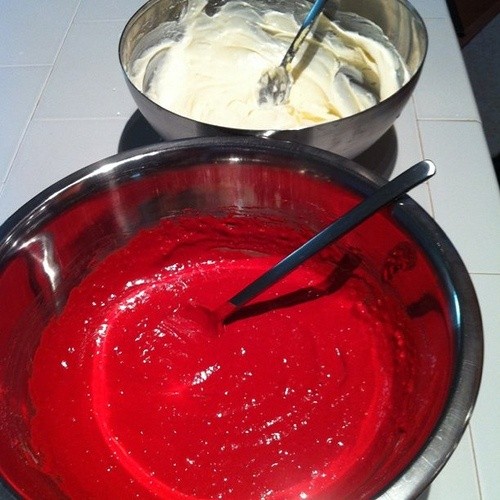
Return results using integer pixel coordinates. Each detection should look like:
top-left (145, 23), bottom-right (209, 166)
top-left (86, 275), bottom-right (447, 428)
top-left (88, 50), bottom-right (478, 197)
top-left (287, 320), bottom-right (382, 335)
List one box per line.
top-left (0, 135), bottom-right (484, 500)
top-left (118, 2), bottom-right (428, 158)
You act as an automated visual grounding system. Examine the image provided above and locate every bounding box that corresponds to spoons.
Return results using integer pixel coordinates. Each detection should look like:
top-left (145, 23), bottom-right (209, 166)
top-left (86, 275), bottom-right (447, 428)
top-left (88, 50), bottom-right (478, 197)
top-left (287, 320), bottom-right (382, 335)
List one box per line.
top-left (256, 0), bottom-right (331, 107)
top-left (192, 159), bottom-right (439, 321)
